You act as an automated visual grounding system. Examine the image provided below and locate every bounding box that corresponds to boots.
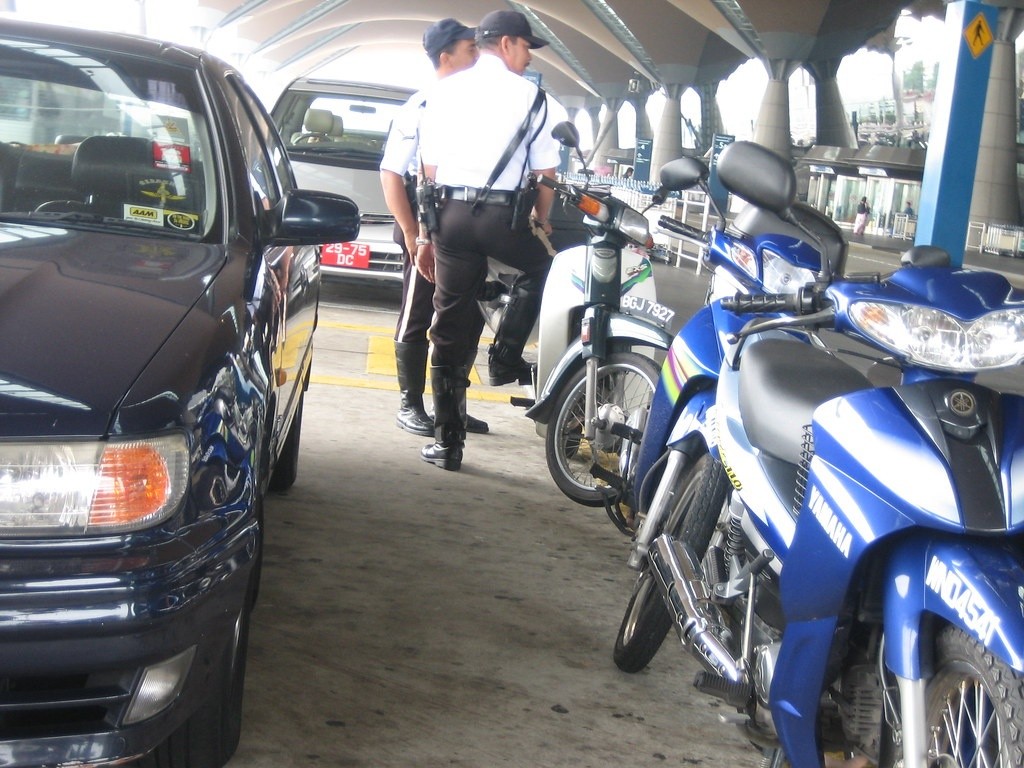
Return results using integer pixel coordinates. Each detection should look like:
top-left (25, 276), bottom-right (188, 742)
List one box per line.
top-left (428, 349), bottom-right (490, 434)
top-left (486, 284), bottom-right (538, 386)
top-left (395, 340), bottom-right (435, 436)
top-left (422, 364), bottom-right (471, 471)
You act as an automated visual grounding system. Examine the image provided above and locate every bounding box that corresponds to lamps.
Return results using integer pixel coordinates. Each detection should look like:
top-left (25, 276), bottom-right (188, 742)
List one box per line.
top-left (628, 71), bottom-right (640, 94)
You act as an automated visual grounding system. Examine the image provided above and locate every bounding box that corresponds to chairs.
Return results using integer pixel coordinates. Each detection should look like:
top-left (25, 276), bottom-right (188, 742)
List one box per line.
top-left (62, 135), bottom-right (182, 226)
top-left (325, 115), bottom-right (344, 143)
top-left (290, 108), bottom-right (333, 143)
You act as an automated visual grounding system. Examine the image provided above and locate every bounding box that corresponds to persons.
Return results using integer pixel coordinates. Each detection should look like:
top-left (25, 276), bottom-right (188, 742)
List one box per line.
top-left (904, 202), bottom-right (913, 215)
top-left (380, 9), bottom-right (561, 471)
top-left (622, 167), bottom-right (634, 179)
top-left (853, 196), bottom-right (870, 236)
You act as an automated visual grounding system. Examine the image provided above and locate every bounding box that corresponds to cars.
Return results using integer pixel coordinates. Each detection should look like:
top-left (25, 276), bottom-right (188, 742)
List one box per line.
top-left (0, 18), bottom-right (362, 768)
top-left (264, 74), bottom-right (415, 290)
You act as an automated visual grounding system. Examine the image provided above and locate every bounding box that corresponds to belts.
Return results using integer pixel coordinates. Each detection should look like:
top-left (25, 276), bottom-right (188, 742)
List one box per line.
top-left (406, 173), bottom-right (417, 184)
top-left (444, 186), bottom-right (518, 206)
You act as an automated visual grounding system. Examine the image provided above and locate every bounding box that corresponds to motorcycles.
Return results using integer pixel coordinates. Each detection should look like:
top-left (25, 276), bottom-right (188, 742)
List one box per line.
top-left (480, 127), bottom-right (710, 508)
top-left (646, 262), bottom-right (1022, 767)
top-left (588, 144), bottom-right (952, 671)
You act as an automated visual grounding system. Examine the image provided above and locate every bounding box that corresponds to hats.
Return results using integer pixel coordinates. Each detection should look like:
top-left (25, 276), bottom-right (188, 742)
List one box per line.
top-left (422, 18), bottom-right (480, 57)
top-left (474, 10), bottom-right (550, 49)
